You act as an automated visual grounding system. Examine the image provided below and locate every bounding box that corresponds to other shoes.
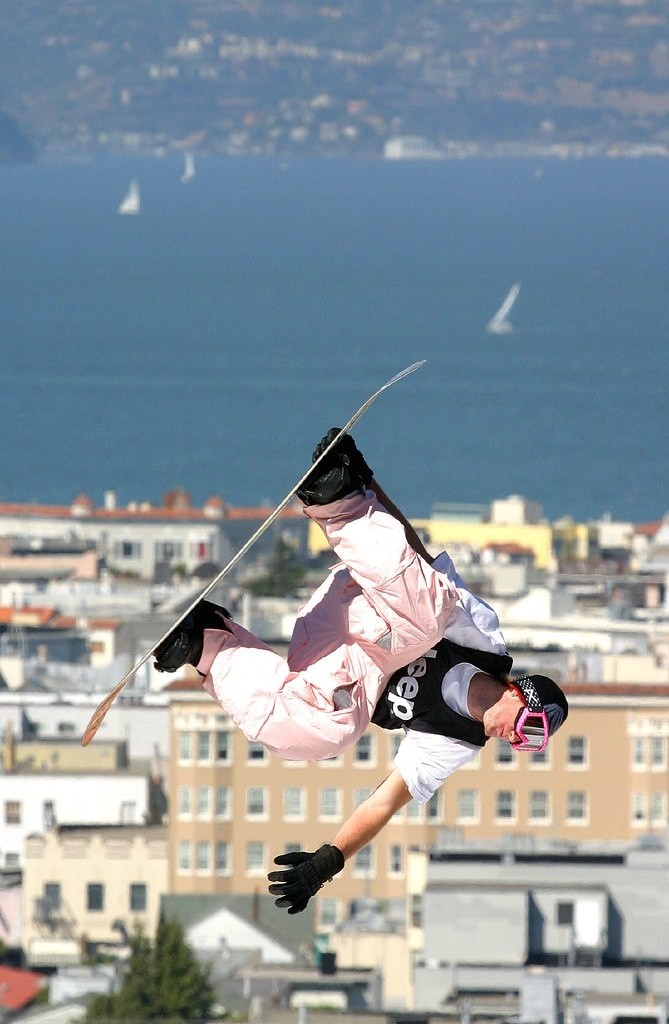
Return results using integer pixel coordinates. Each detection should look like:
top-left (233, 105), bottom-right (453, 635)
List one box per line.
top-left (152, 598), bottom-right (234, 673)
top-left (296, 455), bottom-right (366, 507)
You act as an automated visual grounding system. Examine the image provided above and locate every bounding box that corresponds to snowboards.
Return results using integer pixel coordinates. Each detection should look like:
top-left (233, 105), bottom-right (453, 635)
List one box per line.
top-left (77, 361), bottom-right (425, 750)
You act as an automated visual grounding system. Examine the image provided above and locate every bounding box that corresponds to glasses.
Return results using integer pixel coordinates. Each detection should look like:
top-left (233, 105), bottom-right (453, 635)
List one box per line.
top-left (510, 675), bottom-right (550, 754)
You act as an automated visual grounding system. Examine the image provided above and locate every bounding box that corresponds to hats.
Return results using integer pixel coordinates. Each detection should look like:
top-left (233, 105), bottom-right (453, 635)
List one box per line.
top-left (508, 671), bottom-right (570, 739)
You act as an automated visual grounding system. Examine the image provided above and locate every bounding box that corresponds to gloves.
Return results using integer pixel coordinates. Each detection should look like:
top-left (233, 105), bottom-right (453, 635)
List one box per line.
top-left (265, 842), bottom-right (345, 916)
top-left (310, 426), bottom-right (374, 490)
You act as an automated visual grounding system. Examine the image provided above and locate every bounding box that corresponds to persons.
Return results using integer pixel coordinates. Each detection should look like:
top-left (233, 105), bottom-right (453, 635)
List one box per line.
top-left (153, 428), bottom-right (569, 915)
